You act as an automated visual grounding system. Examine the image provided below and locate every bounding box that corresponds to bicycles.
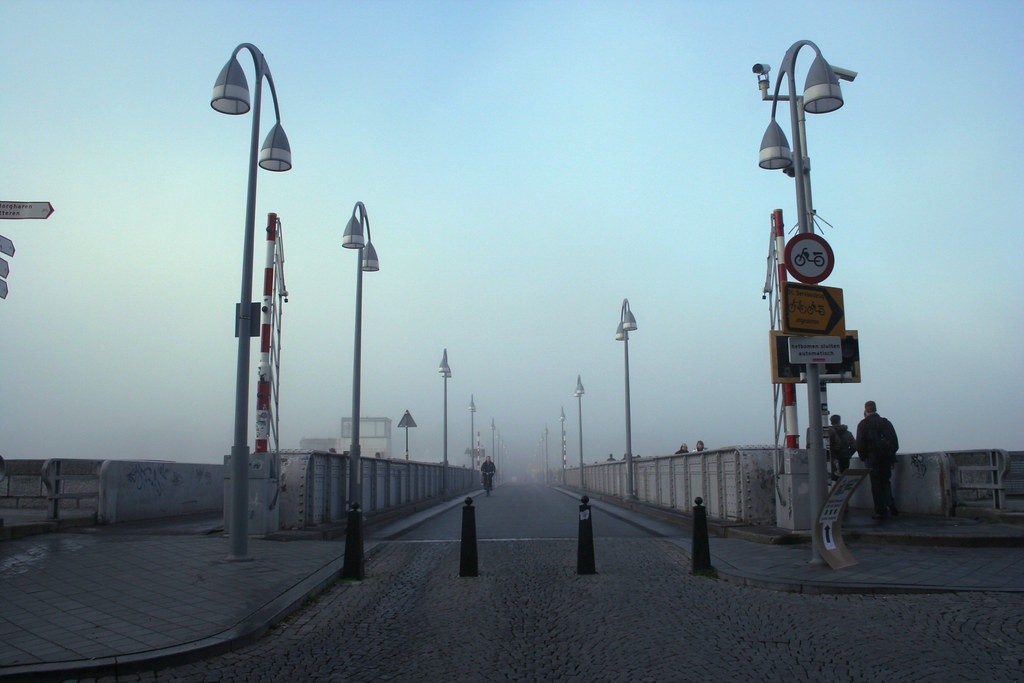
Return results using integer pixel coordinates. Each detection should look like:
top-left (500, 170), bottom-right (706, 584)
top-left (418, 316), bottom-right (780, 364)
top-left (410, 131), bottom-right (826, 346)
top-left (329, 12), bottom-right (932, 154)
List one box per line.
top-left (481, 470), bottom-right (495, 498)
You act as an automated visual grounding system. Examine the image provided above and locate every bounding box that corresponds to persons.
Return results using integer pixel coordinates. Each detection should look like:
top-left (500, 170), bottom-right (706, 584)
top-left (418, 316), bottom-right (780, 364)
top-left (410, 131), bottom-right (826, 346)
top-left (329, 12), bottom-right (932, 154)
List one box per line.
top-left (693, 441), bottom-right (706, 452)
top-left (480, 456), bottom-right (496, 491)
top-left (675, 443), bottom-right (689, 454)
top-left (828, 415), bottom-right (856, 473)
top-left (622, 454), bottom-right (627, 461)
top-left (606, 453), bottom-right (616, 462)
top-left (857, 401), bottom-right (898, 520)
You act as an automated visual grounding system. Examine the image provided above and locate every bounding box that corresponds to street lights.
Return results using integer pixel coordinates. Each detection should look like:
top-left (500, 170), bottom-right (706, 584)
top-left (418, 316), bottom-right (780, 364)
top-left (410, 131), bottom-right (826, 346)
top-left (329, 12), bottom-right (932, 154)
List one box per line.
top-left (207, 41), bottom-right (294, 561)
top-left (756, 39), bottom-right (861, 565)
top-left (489, 418), bottom-right (497, 485)
top-left (341, 201), bottom-right (380, 511)
top-left (467, 394), bottom-right (477, 488)
top-left (543, 423), bottom-right (549, 484)
top-left (574, 374), bottom-right (585, 490)
top-left (437, 348), bottom-right (454, 495)
top-left (559, 406), bottom-right (566, 486)
top-left (614, 298), bottom-right (638, 501)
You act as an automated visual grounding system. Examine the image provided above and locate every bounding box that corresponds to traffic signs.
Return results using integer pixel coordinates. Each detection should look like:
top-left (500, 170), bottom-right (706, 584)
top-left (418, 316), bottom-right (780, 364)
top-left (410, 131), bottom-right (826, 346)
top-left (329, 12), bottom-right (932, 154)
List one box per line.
top-left (0, 200), bottom-right (54, 220)
top-left (0, 257), bottom-right (9, 278)
top-left (0, 234), bottom-right (15, 257)
top-left (0, 278), bottom-right (9, 300)
